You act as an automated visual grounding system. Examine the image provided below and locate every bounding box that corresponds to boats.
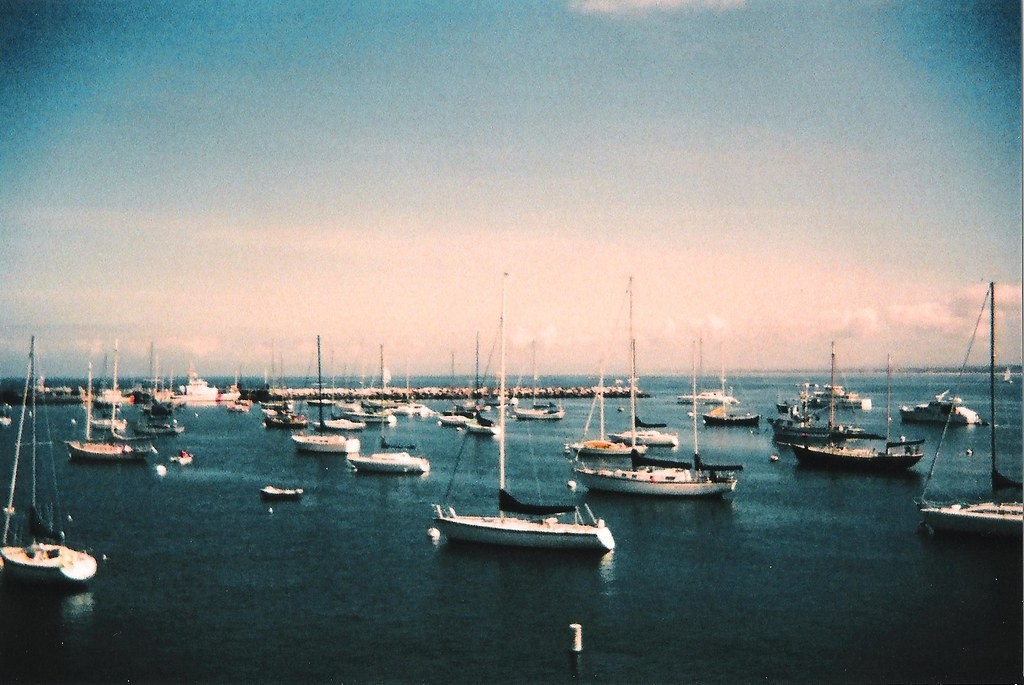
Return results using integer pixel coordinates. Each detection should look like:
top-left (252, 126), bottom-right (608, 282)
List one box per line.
top-left (258, 483), bottom-right (303, 501)
top-left (899, 389), bottom-right (986, 429)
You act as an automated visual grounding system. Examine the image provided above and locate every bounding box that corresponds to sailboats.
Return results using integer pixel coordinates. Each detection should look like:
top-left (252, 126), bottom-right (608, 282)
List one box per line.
top-left (705, 340), bottom-right (762, 431)
top-left (63, 338), bottom-right (157, 462)
top-left (766, 340), bottom-right (890, 454)
top-left (428, 271), bottom-right (618, 552)
top-left (290, 334), bottom-right (363, 455)
top-left (788, 339), bottom-right (929, 471)
top-left (802, 342), bottom-right (873, 411)
top-left (33, 339), bottom-right (253, 440)
top-left (255, 301), bottom-right (567, 445)
top-left (1001, 366), bottom-right (1014, 385)
top-left (344, 344), bottom-right (432, 475)
top-left (913, 282), bottom-right (1024, 530)
top-left (570, 272), bottom-right (742, 499)
top-left (1, 333), bottom-right (98, 589)
